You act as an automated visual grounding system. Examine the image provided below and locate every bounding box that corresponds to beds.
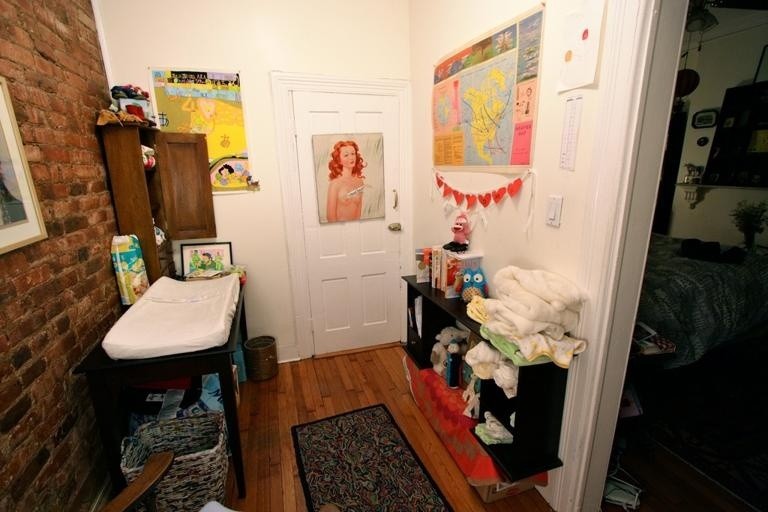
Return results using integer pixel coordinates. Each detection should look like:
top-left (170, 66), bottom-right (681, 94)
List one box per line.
top-left (627, 229), bottom-right (763, 379)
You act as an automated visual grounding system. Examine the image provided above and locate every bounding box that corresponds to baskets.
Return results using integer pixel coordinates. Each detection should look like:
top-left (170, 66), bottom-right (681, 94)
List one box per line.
top-left (121, 410), bottom-right (229, 512)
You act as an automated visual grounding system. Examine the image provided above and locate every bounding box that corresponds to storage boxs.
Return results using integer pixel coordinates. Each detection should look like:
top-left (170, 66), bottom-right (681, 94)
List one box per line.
top-left (471, 476), bottom-right (535, 503)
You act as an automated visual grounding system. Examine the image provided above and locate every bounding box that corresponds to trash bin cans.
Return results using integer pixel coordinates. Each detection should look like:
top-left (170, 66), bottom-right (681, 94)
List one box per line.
top-left (244, 336), bottom-right (278, 381)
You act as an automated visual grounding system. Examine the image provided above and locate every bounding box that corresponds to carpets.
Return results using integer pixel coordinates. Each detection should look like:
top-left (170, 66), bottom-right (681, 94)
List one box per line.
top-left (289, 403), bottom-right (452, 511)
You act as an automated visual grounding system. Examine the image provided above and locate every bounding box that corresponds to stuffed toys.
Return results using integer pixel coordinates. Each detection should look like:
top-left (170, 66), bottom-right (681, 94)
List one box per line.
top-left (444, 209), bottom-right (479, 252)
top-left (428, 266), bottom-right (518, 421)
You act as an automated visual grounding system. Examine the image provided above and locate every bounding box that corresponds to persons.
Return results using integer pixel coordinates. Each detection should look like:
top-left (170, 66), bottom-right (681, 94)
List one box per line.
top-left (325, 140), bottom-right (365, 224)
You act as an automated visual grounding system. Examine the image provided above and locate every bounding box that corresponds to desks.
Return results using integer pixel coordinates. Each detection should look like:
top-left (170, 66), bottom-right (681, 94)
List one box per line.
top-left (73, 278), bottom-right (255, 499)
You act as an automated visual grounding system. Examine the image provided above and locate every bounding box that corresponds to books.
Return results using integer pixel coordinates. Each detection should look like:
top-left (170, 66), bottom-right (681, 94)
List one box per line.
top-left (408, 244), bottom-right (486, 299)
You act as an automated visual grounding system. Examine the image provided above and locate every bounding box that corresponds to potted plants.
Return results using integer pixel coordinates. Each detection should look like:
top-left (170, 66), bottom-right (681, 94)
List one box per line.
top-left (725, 196), bottom-right (766, 244)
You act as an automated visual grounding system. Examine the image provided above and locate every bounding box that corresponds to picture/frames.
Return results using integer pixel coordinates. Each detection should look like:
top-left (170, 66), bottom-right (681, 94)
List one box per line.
top-left (179, 239), bottom-right (233, 278)
top-left (691, 107), bottom-right (721, 128)
top-left (0, 77), bottom-right (53, 255)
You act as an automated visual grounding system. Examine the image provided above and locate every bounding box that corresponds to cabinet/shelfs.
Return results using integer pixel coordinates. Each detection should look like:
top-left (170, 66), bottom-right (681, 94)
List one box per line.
top-left (398, 270), bottom-right (586, 481)
top-left (100, 119), bottom-right (218, 285)
